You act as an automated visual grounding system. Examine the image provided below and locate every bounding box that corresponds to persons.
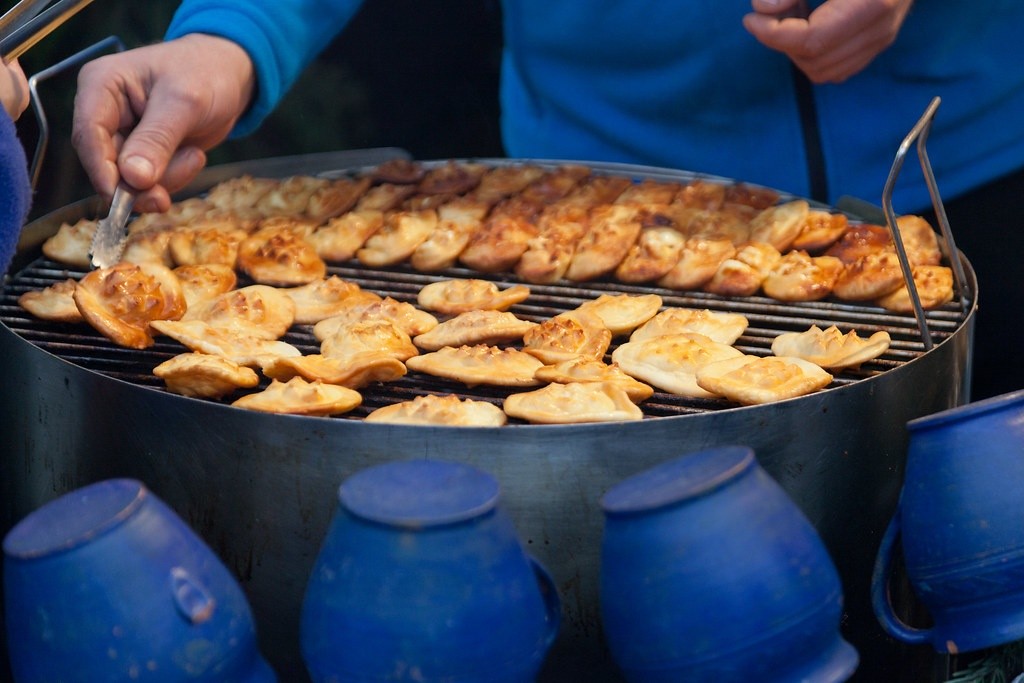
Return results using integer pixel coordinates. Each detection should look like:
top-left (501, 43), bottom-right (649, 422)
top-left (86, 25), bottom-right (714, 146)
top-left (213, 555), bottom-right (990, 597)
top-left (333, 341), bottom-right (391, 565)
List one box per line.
top-left (65, 1), bottom-right (1024, 417)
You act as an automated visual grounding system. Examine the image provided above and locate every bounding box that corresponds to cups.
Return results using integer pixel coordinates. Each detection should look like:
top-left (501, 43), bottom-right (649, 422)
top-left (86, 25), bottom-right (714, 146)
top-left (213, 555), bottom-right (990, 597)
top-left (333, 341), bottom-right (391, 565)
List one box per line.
top-left (4, 476), bottom-right (282, 682)
top-left (601, 442), bottom-right (858, 682)
top-left (870, 387), bottom-right (1024, 652)
top-left (299, 460), bottom-right (563, 683)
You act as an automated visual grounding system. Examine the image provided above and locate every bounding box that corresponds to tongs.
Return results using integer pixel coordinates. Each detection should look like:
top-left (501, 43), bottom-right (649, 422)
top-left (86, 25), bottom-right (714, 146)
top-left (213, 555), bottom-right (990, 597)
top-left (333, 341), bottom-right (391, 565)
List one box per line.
top-left (0, 0), bottom-right (95, 66)
top-left (91, 177), bottom-right (138, 272)
